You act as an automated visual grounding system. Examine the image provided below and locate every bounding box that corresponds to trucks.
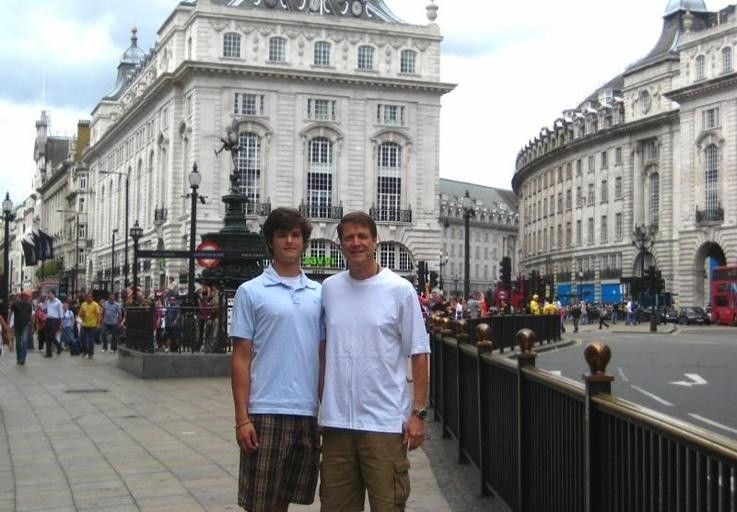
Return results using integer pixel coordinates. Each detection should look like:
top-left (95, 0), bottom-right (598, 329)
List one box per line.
top-left (35, 278), bottom-right (68, 304)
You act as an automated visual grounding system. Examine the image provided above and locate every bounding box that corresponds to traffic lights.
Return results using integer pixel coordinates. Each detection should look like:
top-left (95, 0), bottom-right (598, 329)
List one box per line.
top-left (644, 265), bottom-right (656, 295)
top-left (498, 256), bottom-right (512, 288)
top-left (514, 275), bottom-right (522, 292)
top-left (428, 270), bottom-right (439, 291)
top-left (58, 276), bottom-right (68, 296)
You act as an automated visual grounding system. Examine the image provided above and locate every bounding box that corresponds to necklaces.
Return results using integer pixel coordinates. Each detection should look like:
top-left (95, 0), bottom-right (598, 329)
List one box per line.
top-left (374, 261), bottom-right (380, 276)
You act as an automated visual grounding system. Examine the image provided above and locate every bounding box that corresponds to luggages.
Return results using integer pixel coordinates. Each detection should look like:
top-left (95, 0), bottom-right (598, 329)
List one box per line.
top-left (61, 324), bottom-right (82, 354)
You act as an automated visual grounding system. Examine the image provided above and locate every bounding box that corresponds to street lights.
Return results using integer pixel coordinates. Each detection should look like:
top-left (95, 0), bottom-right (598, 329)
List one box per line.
top-left (440, 247), bottom-right (450, 290)
top-left (0, 189), bottom-right (19, 318)
top-left (460, 188), bottom-right (475, 299)
top-left (98, 169), bottom-right (130, 290)
top-left (179, 160), bottom-right (209, 306)
top-left (54, 208), bottom-right (79, 300)
top-left (129, 216), bottom-right (145, 304)
top-left (630, 223), bottom-right (657, 322)
top-left (450, 268), bottom-right (463, 291)
top-left (110, 228), bottom-right (119, 295)
top-left (577, 269), bottom-right (586, 301)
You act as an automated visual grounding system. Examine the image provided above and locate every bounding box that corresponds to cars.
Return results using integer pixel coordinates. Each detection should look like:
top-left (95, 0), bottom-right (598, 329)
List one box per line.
top-left (588, 301), bottom-right (713, 327)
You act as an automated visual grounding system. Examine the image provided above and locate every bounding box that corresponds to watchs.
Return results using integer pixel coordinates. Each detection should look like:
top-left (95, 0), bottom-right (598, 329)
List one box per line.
top-left (410, 407), bottom-right (429, 420)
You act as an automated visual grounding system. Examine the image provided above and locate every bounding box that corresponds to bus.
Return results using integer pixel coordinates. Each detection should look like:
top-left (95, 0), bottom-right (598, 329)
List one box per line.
top-left (709, 261), bottom-right (737, 328)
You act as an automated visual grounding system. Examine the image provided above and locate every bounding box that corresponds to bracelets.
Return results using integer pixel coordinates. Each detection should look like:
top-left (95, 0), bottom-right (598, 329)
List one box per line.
top-left (233, 422), bottom-right (252, 428)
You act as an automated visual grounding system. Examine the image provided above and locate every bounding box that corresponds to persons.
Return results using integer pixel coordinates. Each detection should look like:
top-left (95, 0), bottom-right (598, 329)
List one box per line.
top-left (0, 279), bottom-right (233, 362)
top-left (225, 207), bottom-right (325, 512)
top-left (314, 212), bottom-right (438, 510)
top-left (399, 271), bottom-right (644, 381)
